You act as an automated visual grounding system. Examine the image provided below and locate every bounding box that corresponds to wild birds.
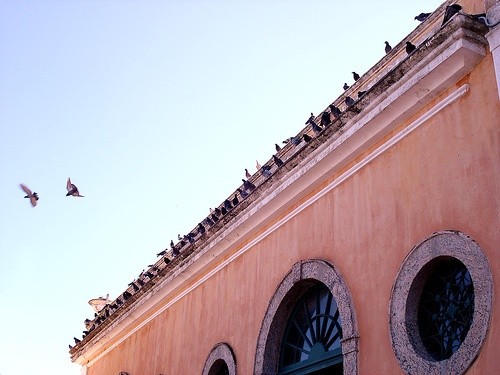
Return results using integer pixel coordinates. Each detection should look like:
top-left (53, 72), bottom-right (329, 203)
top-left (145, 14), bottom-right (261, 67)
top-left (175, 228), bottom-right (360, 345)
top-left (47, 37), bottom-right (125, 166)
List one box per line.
top-left (19, 183), bottom-right (38, 208)
top-left (414, 13), bottom-right (431, 21)
top-left (441, 4), bottom-right (463, 26)
top-left (66, 177), bottom-right (84, 197)
top-left (70, 71), bottom-right (367, 344)
top-left (405, 40), bottom-right (416, 54)
top-left (384, 41), bottom-right (391, 54)
